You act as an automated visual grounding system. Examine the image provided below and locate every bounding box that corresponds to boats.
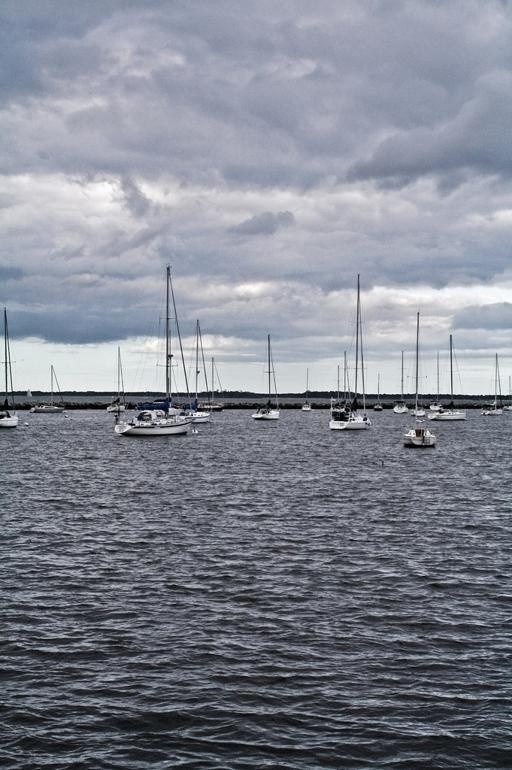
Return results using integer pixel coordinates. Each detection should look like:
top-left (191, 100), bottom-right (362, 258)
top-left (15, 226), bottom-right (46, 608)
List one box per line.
top-left (412, 311), bottom-right (425, 418)
top-left (403, 419), bottom-right (439, 451)
top-left (179, 408), bottom-right (210, 424)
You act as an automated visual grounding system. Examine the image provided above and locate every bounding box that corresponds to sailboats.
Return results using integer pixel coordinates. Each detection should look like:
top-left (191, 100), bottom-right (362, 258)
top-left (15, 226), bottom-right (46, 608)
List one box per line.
top-left (251, 332), bottom-right (282, 421)
top-left (30, 361), bottom-right (70, 414)
top-left (0, 304), bottom-right (20, 431)
top-left (428, 350), bottom-right (447, 411)
top-left (373, 371), bottom-right (383, 413)
top-left (192, 356), bottom-right (230, 410)
top-left (105, 342), bottom-right (131, 415)
top-left (115, 264), bottom-right (193, 438)
top-left (481, 351), bottom-right (506, 416)
top-left (328, 272), bottom-right (372, 430)
top-left (300, 367), bottom-right (315, 413)
top-left (392, 348), bottom-right (410, 414)
top-left (503, 376), bottom-right (512, 412)
top-left (426, 333), bottom-right (468, 420)
